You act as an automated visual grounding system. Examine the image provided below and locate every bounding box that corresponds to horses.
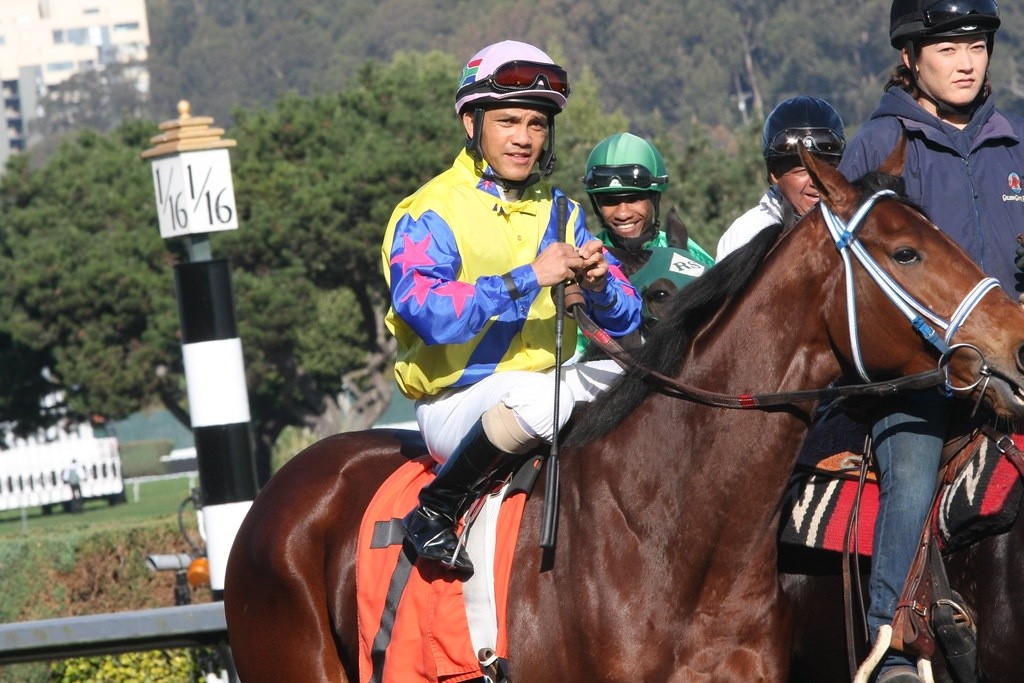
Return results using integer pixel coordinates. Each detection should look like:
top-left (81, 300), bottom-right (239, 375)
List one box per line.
top-left (223, 131), bottom-right (1024, 683)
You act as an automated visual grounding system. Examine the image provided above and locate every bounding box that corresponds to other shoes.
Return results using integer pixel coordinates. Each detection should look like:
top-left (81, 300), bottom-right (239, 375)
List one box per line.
top-left (877, 665), bottom-right (920, 683)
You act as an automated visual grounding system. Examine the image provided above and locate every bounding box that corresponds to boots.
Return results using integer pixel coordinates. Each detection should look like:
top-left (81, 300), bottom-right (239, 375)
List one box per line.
top-left (404, 412), bottom-right (538, 572)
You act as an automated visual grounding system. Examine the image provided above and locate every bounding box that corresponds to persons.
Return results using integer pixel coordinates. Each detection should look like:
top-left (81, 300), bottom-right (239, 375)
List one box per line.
top-left (380, 40), bottom-right (649, 580)
top-left (837, 1), bottom-right (1022, 683)
top-left (715, 96), bottom-right (847, 269)
top-left (563, 132), bottom-right (715, 364)
top-left (0, 402), bottom-right (128, 515)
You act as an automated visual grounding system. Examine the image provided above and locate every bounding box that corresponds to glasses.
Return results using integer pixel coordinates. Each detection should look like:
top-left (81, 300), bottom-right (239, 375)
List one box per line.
top-left (769, 127), bottom-right (847, 156)
top-left (923, 0), bottom-right (1001, 27)
top-left (582, 164), bottom-right (669, 190)
top-left (455, 61), bottom-right (571, 99)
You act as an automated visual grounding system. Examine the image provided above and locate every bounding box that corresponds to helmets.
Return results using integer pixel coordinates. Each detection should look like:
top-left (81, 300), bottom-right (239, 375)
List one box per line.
top-left (762, 96), bottom-right (845, 171)
top-left (454, 40), bottom-right (569, 120)
top-left (890, 0), bottom-right (1001, 51)
top-left (580, 132), bottom-right (670, 198)
